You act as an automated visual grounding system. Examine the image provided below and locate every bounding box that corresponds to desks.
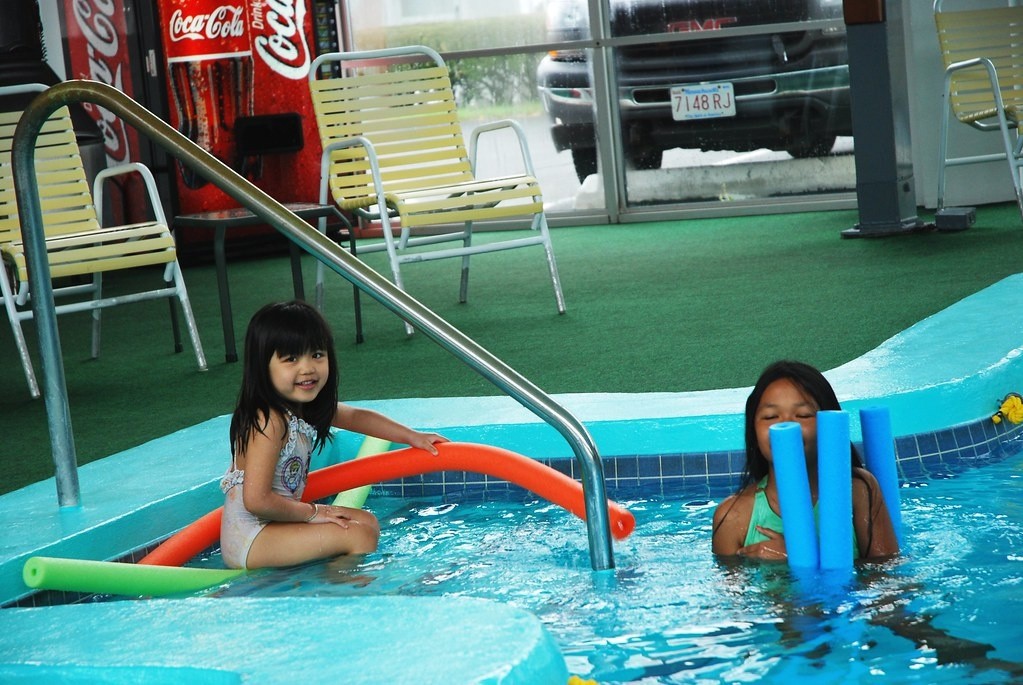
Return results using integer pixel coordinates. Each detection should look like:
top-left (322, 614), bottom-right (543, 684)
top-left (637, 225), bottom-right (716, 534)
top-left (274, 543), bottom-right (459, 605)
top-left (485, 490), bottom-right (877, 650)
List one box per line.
top-left (169, 203), bottom-right (364, 361)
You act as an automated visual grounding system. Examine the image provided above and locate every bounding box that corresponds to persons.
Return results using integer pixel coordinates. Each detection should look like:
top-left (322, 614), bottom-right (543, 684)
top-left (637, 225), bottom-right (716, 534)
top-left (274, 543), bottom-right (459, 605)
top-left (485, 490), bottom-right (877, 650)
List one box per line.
top-left (713, 361), bottom-right (900, 557)
top-left (220, 301), bottom-right (450, 570)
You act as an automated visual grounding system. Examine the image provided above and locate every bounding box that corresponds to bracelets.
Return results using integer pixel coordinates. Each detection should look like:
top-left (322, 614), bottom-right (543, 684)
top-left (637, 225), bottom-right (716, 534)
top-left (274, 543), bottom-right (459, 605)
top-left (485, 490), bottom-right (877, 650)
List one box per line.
top-left (307, 504), bottom-right (318, 522)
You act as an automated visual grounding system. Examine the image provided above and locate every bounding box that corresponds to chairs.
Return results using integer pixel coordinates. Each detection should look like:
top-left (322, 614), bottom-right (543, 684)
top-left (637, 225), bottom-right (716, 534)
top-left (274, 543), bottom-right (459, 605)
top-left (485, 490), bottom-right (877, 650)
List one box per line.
top-left (0, 83), bottom-right (209, 400)
top-left (309, 45), bottom-right (567, 336)
top-left (933, 0), bottom-right (1023, 219)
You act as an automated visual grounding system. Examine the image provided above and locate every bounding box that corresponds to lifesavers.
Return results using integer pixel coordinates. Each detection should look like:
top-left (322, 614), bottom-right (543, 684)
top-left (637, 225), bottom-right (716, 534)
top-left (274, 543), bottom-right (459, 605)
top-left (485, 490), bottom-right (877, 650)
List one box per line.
top-left (773, 402), bottom-right (905, 576)
top-left (23, 434), bottom-right (637, 603)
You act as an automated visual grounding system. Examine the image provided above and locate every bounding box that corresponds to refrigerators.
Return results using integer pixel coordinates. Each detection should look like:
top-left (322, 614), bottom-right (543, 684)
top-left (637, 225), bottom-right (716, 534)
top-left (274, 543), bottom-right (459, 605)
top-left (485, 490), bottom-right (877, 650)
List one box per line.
top-left (57, 0), bottom-right (343, 259)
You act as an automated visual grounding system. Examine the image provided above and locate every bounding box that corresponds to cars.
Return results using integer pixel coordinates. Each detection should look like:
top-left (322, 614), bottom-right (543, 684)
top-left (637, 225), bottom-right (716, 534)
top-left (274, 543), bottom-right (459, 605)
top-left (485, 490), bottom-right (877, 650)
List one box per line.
top-left (534, 1), bottom-right (855, 183)
